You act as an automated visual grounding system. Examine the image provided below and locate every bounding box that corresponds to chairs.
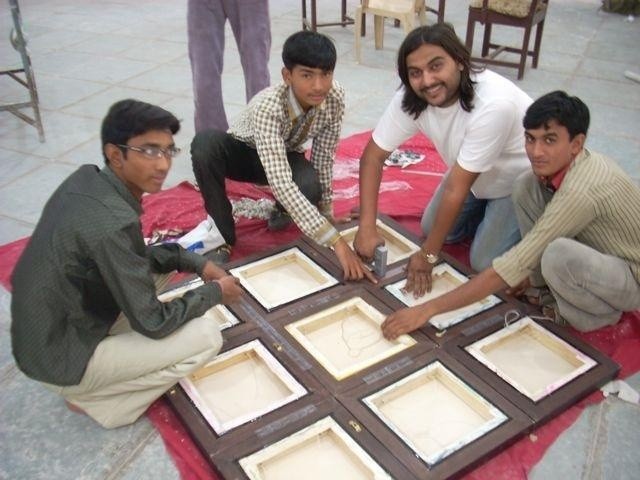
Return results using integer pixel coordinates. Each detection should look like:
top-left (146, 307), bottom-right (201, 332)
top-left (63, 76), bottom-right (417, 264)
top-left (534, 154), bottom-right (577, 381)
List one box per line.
top-left (303, 0), bottom-right (548, 79)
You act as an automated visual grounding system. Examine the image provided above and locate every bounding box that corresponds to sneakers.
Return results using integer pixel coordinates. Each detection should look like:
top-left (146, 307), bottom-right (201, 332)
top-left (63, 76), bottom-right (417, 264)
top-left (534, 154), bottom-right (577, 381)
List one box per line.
top-left (204, 242), bottom-right (232, 265)
top-left (267, 202), bottom-right (291, 231)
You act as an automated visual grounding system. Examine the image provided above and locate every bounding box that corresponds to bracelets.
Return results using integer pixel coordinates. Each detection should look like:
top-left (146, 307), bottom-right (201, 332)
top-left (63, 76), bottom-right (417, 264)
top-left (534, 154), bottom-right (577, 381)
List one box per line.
top-left (211, 279), bottom-right (224, 293)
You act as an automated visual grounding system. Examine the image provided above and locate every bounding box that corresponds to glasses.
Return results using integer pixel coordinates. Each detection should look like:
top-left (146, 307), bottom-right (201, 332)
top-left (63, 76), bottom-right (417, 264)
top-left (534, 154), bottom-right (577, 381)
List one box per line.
top-left (115, 144), bottom-right (182, 158)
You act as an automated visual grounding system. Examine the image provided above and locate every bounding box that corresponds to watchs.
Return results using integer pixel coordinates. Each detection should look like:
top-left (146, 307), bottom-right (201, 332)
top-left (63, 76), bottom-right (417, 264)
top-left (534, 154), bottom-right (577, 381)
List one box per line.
top-left (420, 244), bottom-right (440, 265)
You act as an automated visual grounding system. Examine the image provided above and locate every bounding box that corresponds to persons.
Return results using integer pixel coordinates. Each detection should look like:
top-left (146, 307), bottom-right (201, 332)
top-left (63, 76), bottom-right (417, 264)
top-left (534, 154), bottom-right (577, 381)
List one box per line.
top-left (190, 32), bottom-right (378, 288)
top-left (352, 23), bottom-right (535, 299)
top-left (185, 0), bottom-right (272, 135)
top-left (8, 100), bottom-right (242, 432)
top-left (380, 90), bottom-right (640, 344)
